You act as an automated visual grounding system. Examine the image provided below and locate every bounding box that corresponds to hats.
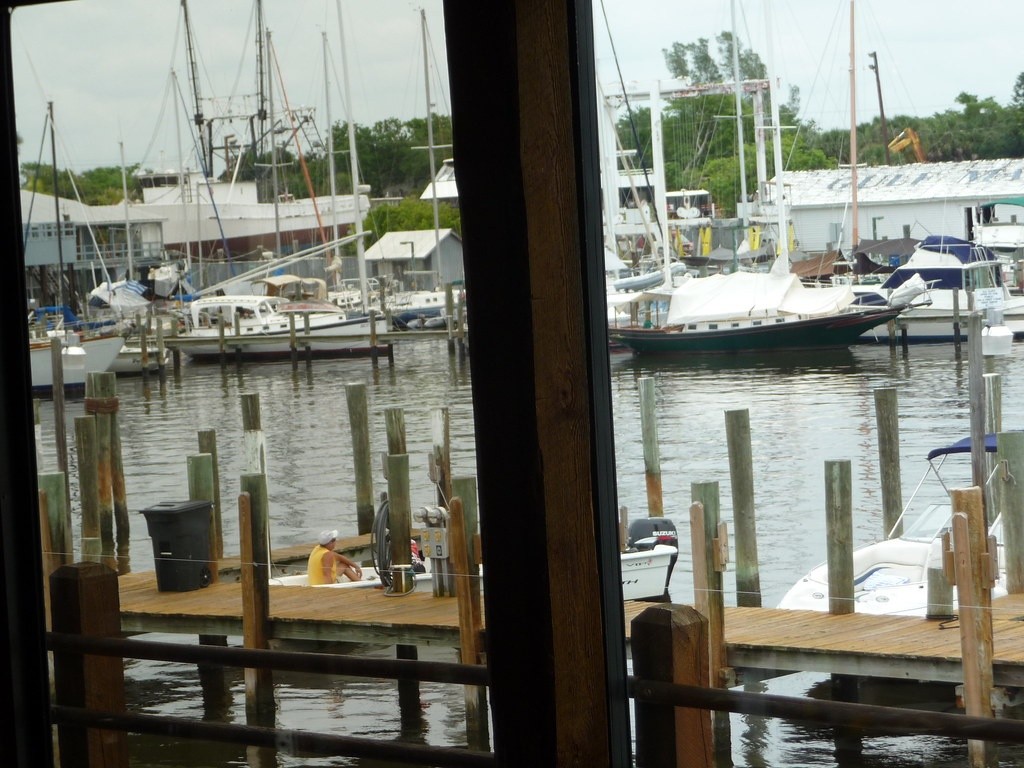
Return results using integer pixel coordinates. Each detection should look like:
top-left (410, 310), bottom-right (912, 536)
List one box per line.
top-left (319, 530), bottom-right (338, 546)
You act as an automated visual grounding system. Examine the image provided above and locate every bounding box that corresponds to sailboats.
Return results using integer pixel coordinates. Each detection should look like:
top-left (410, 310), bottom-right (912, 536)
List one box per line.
top-left (12, 1), bottom-right (470, 396)
top-left (595, 0), bottom-right (1024, 352)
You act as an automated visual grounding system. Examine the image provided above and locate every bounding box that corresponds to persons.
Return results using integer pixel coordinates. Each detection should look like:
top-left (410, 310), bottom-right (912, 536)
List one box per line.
top-left (308, 530), bottom-right (363, 585)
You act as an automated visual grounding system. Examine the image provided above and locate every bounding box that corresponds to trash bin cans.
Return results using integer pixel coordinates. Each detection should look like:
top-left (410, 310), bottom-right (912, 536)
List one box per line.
top-left (139, 499), bottom-right (217, 593)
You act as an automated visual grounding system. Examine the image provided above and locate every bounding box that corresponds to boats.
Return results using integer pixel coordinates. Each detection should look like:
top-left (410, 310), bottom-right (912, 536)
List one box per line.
top-left (266, 500), bottom-right (681, 603)
top-left (776, 372), bottom-right (1008, 616)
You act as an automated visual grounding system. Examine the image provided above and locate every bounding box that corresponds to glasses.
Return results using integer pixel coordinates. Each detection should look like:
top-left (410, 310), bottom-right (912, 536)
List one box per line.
top-left (331, 539), bottom-right (335, 543)
